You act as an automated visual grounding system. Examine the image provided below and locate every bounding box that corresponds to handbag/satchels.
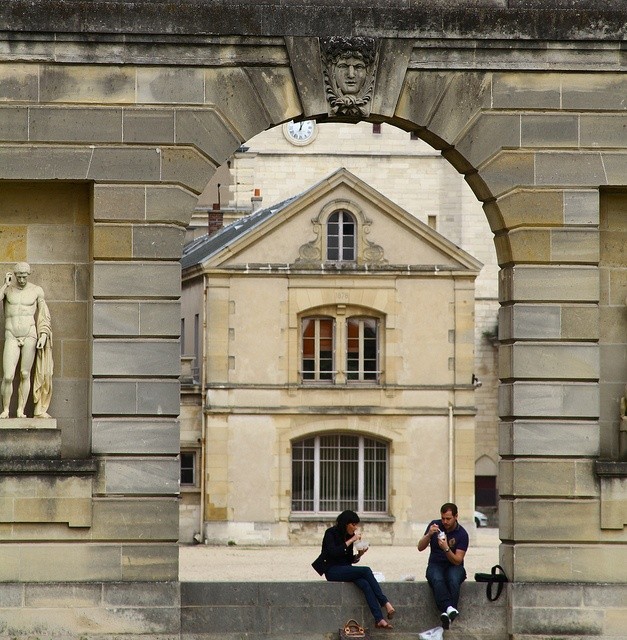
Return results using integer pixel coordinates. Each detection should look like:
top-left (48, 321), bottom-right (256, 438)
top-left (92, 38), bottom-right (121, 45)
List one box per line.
top-left (418, 627), bottom-right (444, 640)
top-left (339, 619), bottom-right (372, 640)
top-left (475, 564), bottom-right (509, 601)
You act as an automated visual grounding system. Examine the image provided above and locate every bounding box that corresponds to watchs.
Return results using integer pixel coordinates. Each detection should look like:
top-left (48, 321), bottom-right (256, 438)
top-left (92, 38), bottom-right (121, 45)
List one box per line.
top-left (444, 546), bottom-right (450, 553)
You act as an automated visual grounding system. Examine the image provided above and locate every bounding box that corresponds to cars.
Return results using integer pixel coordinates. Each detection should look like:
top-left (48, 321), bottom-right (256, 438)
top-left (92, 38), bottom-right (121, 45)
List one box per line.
top-left (475, 510), bottom-right (489, 529)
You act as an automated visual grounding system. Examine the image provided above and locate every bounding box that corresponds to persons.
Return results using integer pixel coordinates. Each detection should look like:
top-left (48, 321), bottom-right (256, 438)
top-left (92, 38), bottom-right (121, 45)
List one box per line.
top-left (323, 36), bottom-right (380, 117)
top-left (418, 503), bottom-right (468, 630)
top-left (0, 261), bottom-right (47, 418)
top-left (311, 510), bottom-right (396, 629)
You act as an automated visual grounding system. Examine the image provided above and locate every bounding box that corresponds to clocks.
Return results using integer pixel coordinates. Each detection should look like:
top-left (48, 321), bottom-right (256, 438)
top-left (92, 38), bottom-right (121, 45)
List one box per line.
top-left (282, 118), bottom-right (318, 146)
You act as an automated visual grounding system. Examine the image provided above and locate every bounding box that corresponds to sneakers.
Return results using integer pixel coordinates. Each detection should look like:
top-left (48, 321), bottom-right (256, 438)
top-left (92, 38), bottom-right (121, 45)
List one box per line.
top-left (447, 605), bottom-right (459, 620)
top-left (441, 613), bottom-right (451, 630)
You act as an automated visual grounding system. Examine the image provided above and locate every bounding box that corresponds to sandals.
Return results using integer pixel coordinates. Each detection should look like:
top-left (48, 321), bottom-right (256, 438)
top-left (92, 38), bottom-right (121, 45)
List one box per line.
top-left (376, 622), bottom-right (393, 629)
top-left (387, 609), bottom-right (395, 619)
